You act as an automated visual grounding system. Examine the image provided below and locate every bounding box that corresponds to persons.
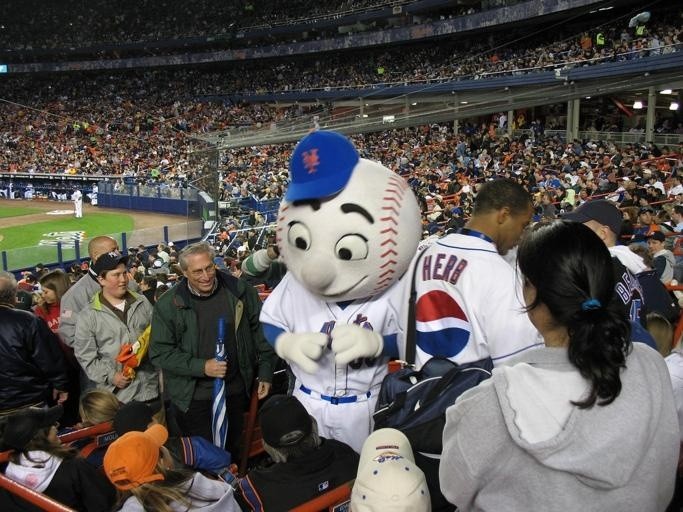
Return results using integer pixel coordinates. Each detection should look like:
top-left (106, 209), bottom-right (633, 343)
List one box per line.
top-left (0, 1), bottom-right (682, 511)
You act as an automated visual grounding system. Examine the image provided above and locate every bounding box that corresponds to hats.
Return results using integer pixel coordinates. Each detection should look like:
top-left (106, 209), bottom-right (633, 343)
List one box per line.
top-left (558, 198), bottom-right (624, 236)
top-left (111, 399), bottom-right (162, 437)
top-left (1, 403), bottom-right (65, 452)
top-left (103, 423), bottom-right (169, 491)
top-left (350, 429), bottom-right (432, 512)
top-left (257, 394), bottom-right (312, 449)
top-left (644, 231), bottom-right (665, 242)
top-left (87, 251), bottom-right (129, 281)
top-left (285, 130), bottom-right (357, 205)
top-left (637, 207), bottom-right (654, 216)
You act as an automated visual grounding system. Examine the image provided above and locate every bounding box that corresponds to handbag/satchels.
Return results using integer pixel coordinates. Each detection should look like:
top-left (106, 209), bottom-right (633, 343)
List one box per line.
top-left (373, 357), bottom-right (494, 511)
